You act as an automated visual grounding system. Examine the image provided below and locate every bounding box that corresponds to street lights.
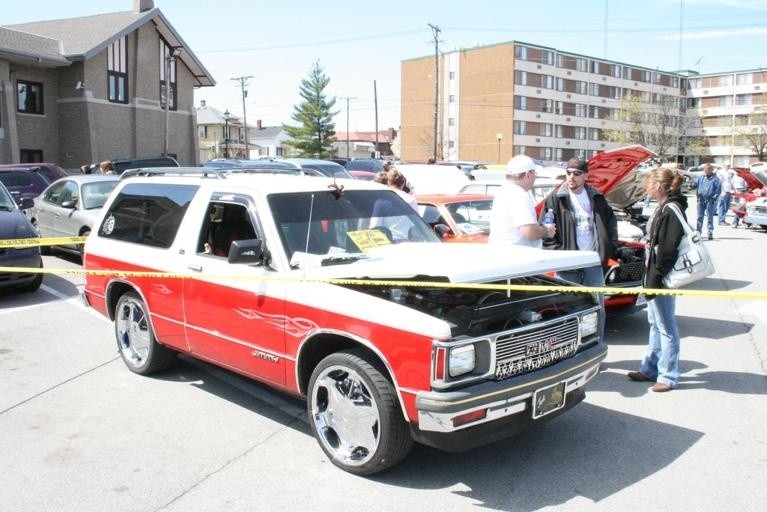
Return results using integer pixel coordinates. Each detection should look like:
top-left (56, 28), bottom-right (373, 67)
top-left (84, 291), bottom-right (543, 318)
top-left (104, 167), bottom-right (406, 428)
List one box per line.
top-left (162, 46), bottom-right (181, 156)
top-left (497, 133), bottom-right (502, 164)
top-left (222, 108), bottom-right (230, 160)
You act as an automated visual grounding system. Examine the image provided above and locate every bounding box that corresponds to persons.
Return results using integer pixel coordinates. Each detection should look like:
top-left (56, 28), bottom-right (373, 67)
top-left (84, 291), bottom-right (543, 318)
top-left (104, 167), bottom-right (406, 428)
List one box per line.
top-left (488, 155), bottom-right (557, 247)
top-left (628, 167), bottom-right (688, 392)
top-left (688, 164), bottom-right (722, 240)
top-left (538, 157), bottom-right (617, 341)
top-left (95, 162), bottom-right (118, 176)
top-left (714, 162), bottom-right (748, 228)
top-left (357, 161), bottom-right (420, 241)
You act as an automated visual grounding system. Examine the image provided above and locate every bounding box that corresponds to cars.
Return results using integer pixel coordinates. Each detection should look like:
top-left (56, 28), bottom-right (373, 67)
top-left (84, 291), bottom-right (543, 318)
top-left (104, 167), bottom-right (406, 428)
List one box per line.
top-left (122, 157), bottom-right (766, 337)
top-left (83, 155), bottom-right (181, 176)
top-left (0, 180), bottom-right (45, 294)
top-left (21, 174), bottom-right (122, 265)
top-left (0, 162), bottom-right (70, 187)
top-left (0, 167), bottom-right (53, 203)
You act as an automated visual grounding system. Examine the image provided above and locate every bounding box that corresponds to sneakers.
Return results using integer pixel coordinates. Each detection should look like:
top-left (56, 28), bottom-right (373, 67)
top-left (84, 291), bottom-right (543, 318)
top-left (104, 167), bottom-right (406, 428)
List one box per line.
top-left (652, 381), bottom-right (670, 392)
top-left (628, 371), bottom-right (646, 381)
top-left (719, 221), bottom-right (730, 226)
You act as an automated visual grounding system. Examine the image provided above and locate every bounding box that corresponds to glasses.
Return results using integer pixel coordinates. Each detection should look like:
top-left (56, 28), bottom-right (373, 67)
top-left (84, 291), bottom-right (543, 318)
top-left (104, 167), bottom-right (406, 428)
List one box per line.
top-left (566, 170), bottom-right (582, 176)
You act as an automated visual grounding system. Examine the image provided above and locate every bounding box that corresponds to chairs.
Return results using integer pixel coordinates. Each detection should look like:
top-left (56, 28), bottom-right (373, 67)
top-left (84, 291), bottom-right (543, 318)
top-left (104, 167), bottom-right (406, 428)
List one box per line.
top-left (211, 220), bottom-right (255, 257)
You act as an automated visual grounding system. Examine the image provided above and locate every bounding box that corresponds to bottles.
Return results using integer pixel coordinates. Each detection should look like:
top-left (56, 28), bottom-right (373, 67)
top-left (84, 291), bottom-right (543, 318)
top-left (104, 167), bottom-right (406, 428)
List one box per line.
top-left (543, 208), bottom-right (554, 224)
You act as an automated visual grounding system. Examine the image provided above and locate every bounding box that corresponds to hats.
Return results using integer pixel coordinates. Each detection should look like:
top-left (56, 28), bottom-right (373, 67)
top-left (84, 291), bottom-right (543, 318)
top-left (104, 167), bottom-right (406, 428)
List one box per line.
top-left (567, 156), bottom-right (588, 172)
top-left (506, 154), bottom-right (542, 175)
top-left (724, 160), bottom-right (731, 166)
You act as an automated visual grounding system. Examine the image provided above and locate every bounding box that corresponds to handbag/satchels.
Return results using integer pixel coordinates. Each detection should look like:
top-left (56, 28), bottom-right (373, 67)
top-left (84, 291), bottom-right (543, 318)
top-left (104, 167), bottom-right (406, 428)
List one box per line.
top-left (654, 204), bottom-right (714, 289)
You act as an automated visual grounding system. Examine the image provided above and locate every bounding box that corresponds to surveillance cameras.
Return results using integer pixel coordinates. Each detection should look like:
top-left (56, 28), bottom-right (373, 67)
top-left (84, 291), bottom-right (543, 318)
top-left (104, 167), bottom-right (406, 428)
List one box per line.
top-left (75, 81), bottom-right (82, 89)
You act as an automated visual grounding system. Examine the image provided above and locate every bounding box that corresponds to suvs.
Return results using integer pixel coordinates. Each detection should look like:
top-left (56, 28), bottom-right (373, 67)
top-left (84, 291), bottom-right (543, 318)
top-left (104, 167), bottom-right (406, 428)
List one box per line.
top-left (81, 166), bottom-right (607, 480)
